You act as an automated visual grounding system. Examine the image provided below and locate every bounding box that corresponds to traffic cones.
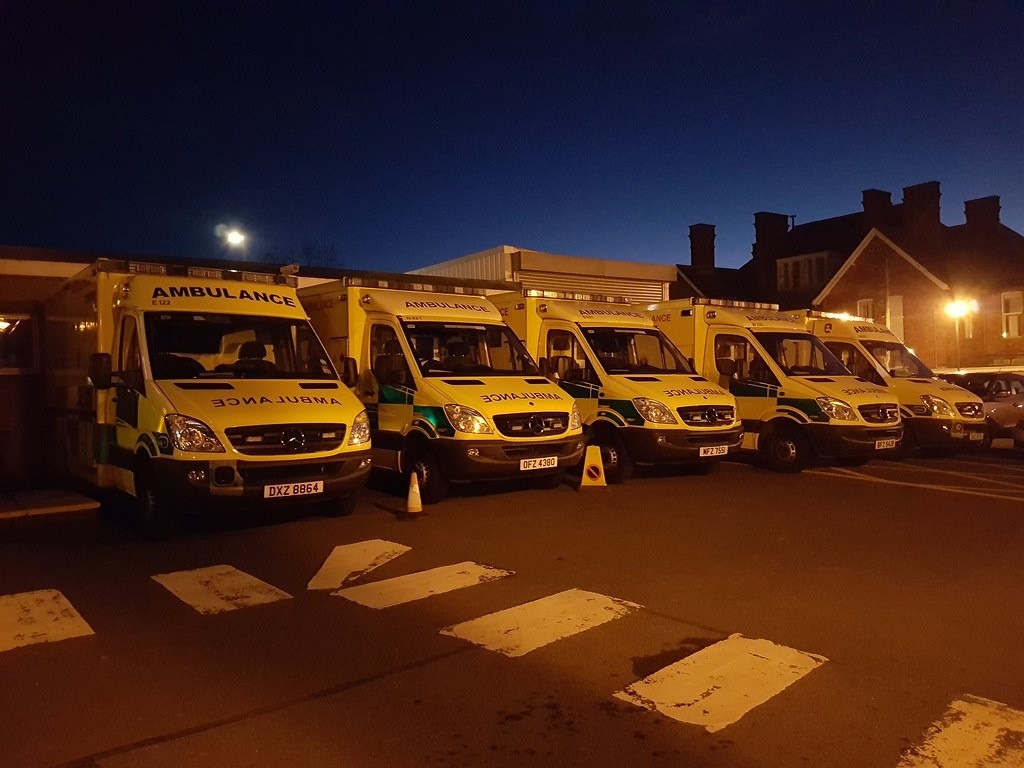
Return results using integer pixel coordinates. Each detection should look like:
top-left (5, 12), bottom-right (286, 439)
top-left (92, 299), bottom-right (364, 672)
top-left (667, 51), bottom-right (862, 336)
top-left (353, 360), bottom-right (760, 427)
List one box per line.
top-left (579, 446), bottom-right (608, 488)
top-left (400, 472), bottom-right (428, 522)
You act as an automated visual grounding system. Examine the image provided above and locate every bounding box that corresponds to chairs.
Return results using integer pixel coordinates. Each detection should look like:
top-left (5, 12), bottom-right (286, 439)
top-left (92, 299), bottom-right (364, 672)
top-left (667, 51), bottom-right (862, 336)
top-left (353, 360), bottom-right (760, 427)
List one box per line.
top-left (716, 344), bottom-right (736, 375)
top-left (374, 340), bottom-right (406, 383)
top-left (151, 332), bottom-right (183, 378)
top-left (599, 336), bottom-right (624, 368)
top-left (551, 336), bottom-right (578, 380)
top-left (233, 340), bottom-right (282, 379)
top-left (444, 341), bottom-right (477, 369)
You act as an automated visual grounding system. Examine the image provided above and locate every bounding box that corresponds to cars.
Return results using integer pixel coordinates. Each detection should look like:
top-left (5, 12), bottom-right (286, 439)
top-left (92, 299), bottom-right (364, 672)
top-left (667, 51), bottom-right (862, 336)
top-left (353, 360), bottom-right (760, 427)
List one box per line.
top-left (955, 372), bottom-right (1024, 448)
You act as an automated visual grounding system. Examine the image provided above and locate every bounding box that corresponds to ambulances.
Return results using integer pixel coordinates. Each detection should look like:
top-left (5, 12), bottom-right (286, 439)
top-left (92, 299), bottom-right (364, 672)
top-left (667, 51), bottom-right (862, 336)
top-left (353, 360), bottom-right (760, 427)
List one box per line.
top-left (606, 297), bottom-right (905, 472)
top-left (734, 307), bottom-right (986, 457)
top-left (418, 290), bottom-right (742, 479)
top-left (220, 276), bottom-right (587, 503)
top-left (29, 259), bottom-right (375, 527)
